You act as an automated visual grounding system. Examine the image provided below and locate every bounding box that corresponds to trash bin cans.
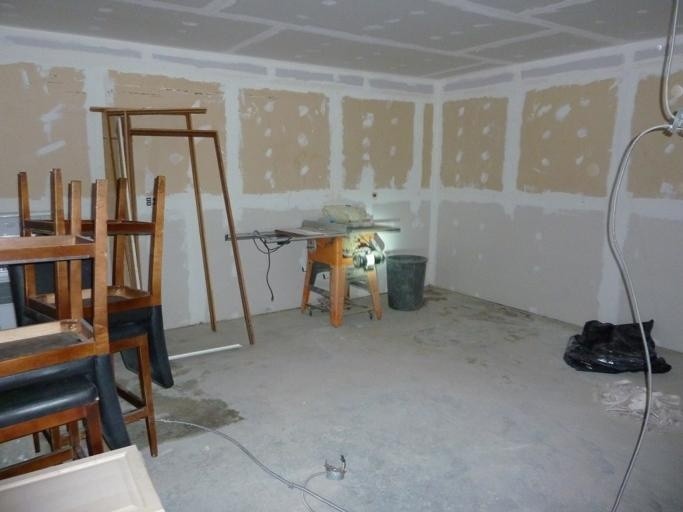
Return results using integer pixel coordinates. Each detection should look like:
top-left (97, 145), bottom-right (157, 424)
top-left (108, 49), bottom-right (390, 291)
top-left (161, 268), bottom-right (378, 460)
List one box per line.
top-left (387, 254), bottom-right (428, 312)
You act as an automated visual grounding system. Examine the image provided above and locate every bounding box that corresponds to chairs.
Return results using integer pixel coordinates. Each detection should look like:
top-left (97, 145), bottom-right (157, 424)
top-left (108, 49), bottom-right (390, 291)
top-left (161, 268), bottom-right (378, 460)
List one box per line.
top-left (0, 167), bottom-right (176, 479)
